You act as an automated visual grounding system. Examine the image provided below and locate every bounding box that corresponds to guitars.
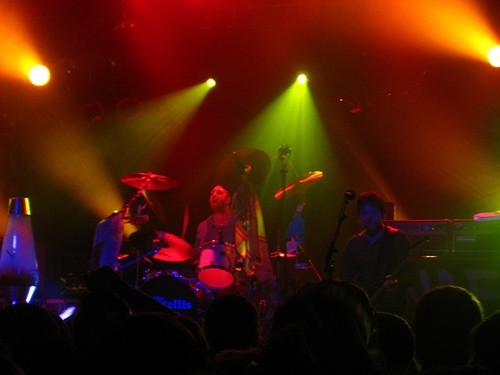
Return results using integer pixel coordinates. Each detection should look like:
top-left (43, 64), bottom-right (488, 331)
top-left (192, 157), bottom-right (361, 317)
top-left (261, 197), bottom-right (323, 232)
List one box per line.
top-left (371, 235), bottom-right (432, 304)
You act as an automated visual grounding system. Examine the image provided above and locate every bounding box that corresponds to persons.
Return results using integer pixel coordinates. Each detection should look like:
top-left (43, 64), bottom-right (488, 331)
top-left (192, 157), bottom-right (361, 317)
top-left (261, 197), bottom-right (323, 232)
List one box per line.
top-left (194, 183), bottom-right (247, 249)
top-left (0, 263), bottom-right (500, 375)
top-left (342, 192), bottom-right (412, 316)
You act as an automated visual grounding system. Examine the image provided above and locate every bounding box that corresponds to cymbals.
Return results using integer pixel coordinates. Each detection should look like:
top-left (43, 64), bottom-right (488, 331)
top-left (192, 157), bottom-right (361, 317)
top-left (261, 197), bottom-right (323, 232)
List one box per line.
top-left (118, 171), bottom-right (177, 190)
top-left (274, 170), bottom-right (324, 201)
top-left (271, 252), bottom-right (297, 258)
top-left (128, 229), bottom-right (195, 263)
top-left (215, 148), bottom-right (272, 193)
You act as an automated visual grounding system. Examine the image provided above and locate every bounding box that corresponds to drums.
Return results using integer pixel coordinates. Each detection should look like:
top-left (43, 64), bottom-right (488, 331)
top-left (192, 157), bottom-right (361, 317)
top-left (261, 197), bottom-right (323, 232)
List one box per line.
top-left (135, 269), bottom-right (215, 335)
top-left (197, 240), bottom-right (236, 288)
